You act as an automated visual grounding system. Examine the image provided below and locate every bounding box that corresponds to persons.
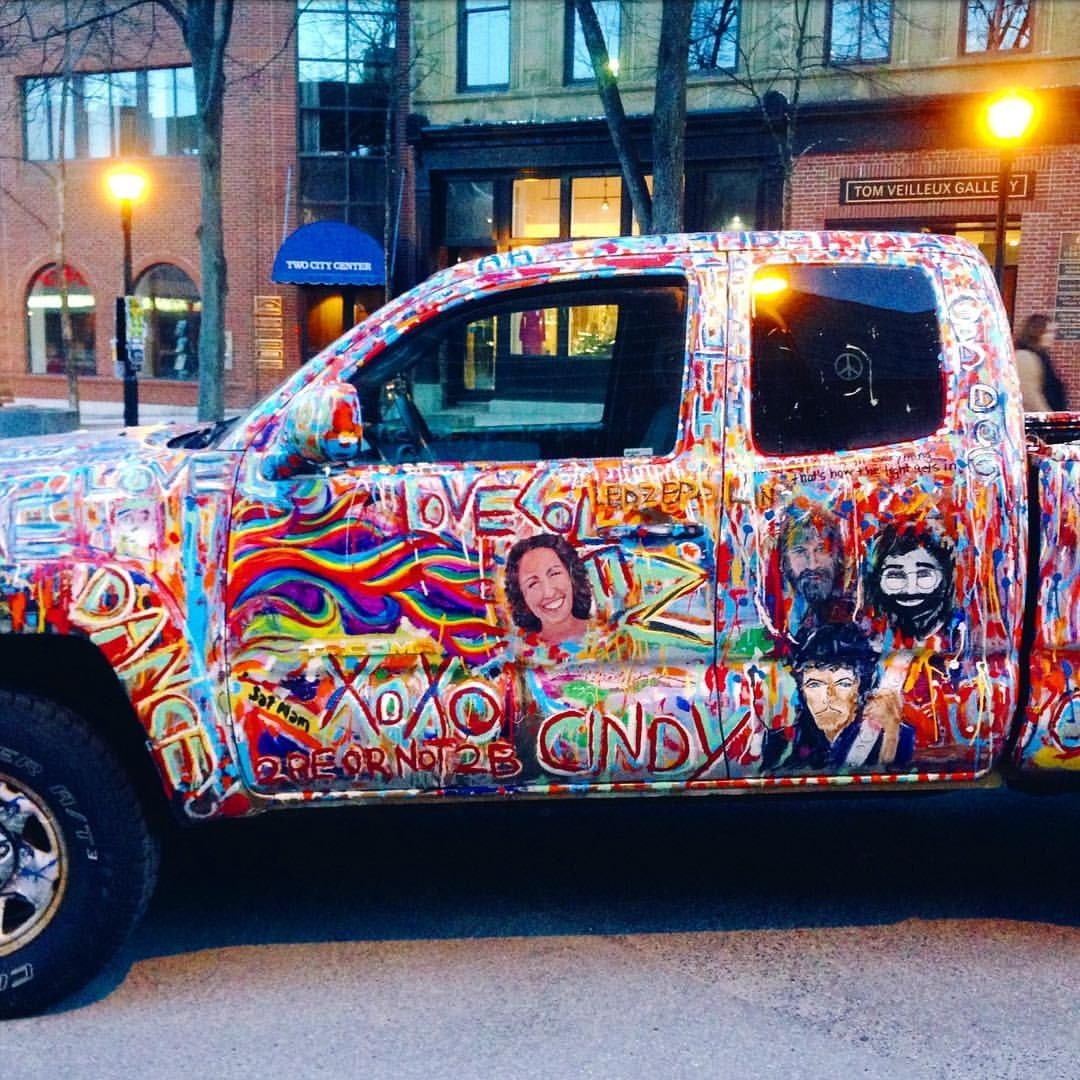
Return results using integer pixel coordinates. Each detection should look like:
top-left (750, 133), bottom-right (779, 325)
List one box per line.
top-left (1014, 312), bottom-right (1069, 412)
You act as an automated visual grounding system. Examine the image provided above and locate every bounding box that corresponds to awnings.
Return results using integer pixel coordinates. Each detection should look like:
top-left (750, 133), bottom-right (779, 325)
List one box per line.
top-left (272, 220), bottom-right (393, 289)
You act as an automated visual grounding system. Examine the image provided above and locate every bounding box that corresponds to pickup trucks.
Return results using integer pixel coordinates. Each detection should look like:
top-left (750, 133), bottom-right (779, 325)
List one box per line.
top-left (1, 229), bottom-right (1080, 1014)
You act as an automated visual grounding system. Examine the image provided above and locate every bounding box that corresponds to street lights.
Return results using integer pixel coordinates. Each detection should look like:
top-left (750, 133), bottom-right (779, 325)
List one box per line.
top-left (102, 164), bottom-right (150, 425)
top-left (978, 85), bottom-right (1043, 292)
